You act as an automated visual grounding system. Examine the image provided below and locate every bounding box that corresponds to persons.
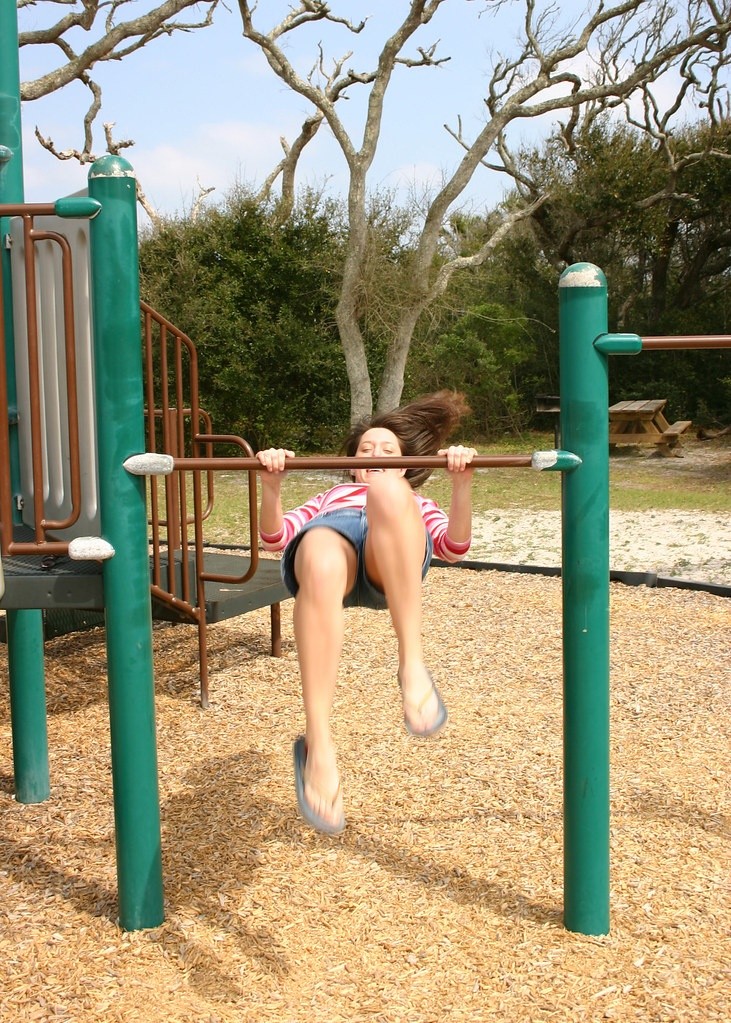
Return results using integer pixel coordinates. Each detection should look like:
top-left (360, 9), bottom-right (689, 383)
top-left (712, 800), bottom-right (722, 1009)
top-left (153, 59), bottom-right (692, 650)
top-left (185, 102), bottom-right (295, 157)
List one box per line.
top-left (255, 389), bottom-right (479, 833)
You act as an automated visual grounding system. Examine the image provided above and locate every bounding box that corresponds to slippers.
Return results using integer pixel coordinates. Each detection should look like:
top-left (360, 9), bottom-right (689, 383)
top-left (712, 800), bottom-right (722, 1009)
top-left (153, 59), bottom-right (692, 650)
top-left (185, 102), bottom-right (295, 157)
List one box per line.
top-left (294, 734), bottom-right (346, 833)
top-left (398, 666), bottom-right (448, 736)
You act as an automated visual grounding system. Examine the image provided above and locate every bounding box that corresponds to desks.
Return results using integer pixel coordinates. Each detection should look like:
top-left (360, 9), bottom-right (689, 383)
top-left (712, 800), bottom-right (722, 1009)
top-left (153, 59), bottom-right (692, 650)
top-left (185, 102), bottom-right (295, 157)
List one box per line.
top-left (608, 399), bottom-right (684, 458)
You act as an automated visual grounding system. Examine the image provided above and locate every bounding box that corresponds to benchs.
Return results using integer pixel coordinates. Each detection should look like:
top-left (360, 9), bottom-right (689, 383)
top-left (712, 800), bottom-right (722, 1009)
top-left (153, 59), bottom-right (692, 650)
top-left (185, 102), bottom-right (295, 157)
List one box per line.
top-left (661, 421), bottom-right (692, 457)
